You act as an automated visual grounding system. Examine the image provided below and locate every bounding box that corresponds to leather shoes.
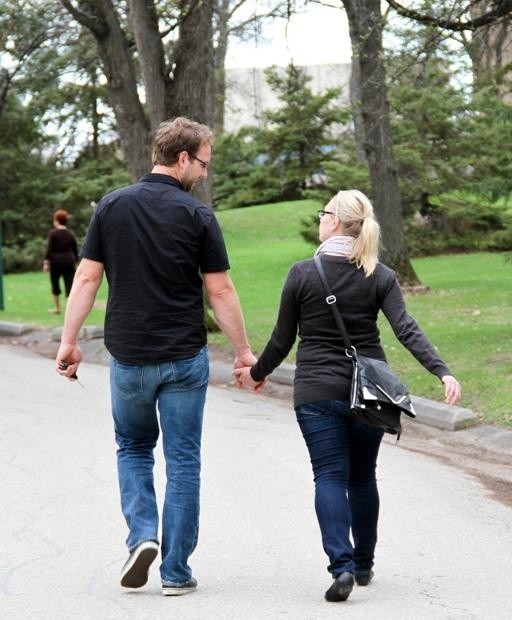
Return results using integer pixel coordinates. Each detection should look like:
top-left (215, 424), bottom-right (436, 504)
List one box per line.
top-left (326, 571), bottom-right (373, 602)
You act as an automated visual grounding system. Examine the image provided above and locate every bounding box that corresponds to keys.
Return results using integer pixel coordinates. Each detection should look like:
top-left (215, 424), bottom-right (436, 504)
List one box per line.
top-left (71, 373), bottom-right (84, 388)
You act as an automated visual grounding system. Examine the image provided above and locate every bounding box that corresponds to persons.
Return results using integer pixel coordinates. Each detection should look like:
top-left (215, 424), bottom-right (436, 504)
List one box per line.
top-left (44, 209), bottom-right (81, 315)
top-left (56, 118), bottom-right (266, 597)
top-left (231, 185), bottom-right (461, 601)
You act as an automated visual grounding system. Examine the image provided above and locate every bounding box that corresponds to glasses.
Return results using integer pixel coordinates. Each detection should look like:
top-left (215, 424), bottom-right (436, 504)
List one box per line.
top-left (318, 211), bottom-right (335, 217)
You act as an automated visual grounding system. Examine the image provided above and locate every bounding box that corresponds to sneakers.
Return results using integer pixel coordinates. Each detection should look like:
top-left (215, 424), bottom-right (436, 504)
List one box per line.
top-left (162, 578), bottom-right (197, 595)
top-left (120, 540), bottom-right (159, 587)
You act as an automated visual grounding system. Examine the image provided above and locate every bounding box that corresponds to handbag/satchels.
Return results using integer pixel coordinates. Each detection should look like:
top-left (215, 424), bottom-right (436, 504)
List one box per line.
top-left (350, 355), bottom-right (416, 440)
